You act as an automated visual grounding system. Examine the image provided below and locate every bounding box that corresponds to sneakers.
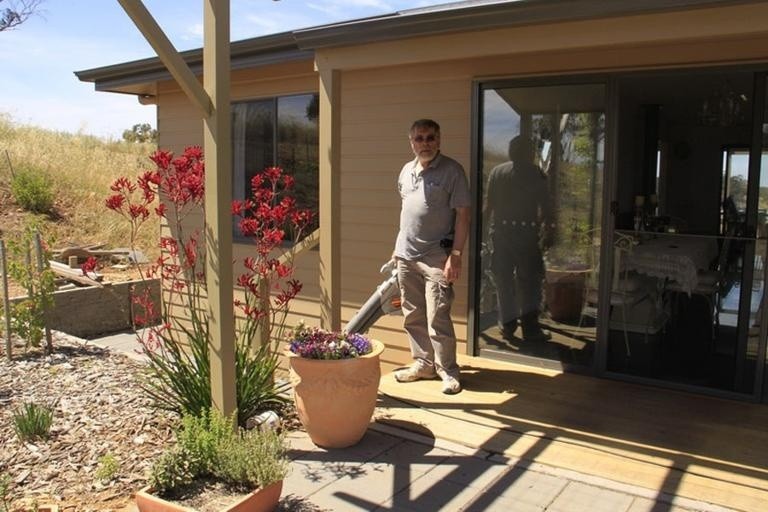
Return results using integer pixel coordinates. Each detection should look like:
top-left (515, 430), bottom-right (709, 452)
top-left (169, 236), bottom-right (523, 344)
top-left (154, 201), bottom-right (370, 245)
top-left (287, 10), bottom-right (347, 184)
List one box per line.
top-left (442, 374), bottom-right (461, 394)
top-left (497, 318), bottom-right (519, 334)
top-left (524, 329), bottom-right (552, 344)
top-left (394, 368), bottom-right (436, 382)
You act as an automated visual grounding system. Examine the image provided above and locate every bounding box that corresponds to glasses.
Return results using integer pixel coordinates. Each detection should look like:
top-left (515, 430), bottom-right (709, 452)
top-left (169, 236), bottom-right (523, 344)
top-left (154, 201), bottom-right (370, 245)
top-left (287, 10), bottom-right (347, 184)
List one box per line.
top-left (413, 134), bottom-right (434, 142)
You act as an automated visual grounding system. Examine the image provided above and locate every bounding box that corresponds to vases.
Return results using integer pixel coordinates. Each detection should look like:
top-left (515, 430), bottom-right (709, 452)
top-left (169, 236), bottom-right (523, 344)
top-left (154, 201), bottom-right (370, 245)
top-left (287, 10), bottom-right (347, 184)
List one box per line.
top-left (545, 263), bottom-right (593, 321)
top-left (283, 340), bottom-right (387, 449)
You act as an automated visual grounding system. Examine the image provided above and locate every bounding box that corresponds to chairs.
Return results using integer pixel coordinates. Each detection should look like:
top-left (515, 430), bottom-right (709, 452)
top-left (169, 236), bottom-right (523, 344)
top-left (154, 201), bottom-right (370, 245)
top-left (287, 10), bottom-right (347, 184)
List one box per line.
top-left (573, 221), bottom-right (736, 358)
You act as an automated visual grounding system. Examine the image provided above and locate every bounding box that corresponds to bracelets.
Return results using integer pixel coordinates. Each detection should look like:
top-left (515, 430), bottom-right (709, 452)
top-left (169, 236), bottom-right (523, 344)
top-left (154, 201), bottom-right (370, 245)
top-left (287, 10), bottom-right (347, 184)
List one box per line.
top-left (451, 249), bottom-right (462, 257)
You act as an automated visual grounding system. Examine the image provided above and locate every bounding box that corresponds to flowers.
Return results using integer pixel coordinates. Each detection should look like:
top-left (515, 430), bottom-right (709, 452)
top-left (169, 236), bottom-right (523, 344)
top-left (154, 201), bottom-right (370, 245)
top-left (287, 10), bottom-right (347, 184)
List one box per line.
top-left (282, 320), bottom-right (373, 360)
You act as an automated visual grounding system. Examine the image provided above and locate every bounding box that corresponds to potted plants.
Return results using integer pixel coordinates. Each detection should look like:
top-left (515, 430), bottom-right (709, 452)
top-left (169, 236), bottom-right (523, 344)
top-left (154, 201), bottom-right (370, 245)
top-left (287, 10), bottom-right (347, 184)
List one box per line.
top-left (135, 407), bottom-right (289, 512)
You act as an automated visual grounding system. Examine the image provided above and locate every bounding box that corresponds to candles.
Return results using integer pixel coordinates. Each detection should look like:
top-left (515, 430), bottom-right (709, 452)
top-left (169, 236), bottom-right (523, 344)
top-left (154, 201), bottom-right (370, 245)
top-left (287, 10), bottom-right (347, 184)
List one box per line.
top-left (634, 194), bottom-right (657, 208)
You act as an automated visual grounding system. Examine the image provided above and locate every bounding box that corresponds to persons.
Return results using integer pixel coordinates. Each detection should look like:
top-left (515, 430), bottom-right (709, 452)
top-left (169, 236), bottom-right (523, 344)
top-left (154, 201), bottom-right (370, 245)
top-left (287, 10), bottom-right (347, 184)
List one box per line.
top-left (390, 119), bottom-right (473, 396)
top-left (484, 133), bottom-right (552, 344)
